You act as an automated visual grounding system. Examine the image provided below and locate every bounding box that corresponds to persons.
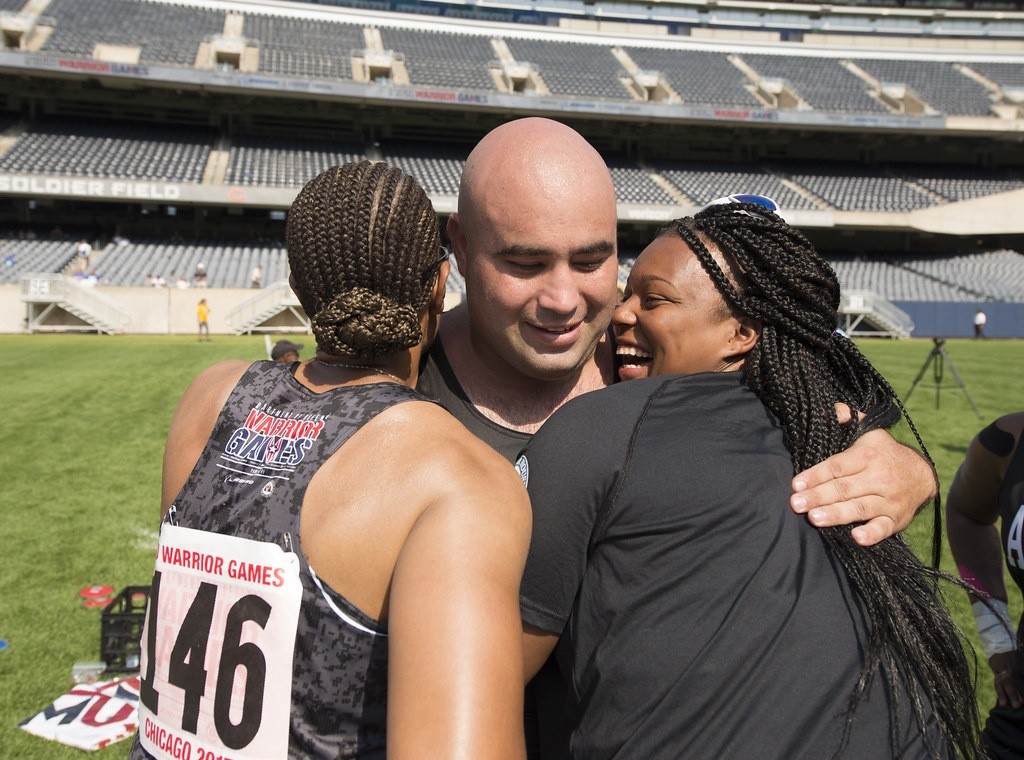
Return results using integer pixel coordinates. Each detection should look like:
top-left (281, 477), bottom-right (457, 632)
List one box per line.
top-left (523, 194), bottom-right (982, 759)
top-left (252, 265), bottom-right (261, 289)
top-left (127, 116), bottom-right (533, 760)
top-left (146, 262), bottom-right (207, 288)
top-left (270, 340), bottom-right (304, 362)
top-left (946, 411), bottom-right (1024, 759)
top-left (974, 309), bottom-right (986, 339)
top-left (197, 299), bottom-right (210, 342)
top-left (79, 239), bottom-right (92, 272)
top-left (415, 118), bottom-right (625, 467)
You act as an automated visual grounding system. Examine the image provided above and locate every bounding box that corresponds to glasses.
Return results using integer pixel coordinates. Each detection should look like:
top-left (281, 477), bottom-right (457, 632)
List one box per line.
top-left (421, 247), bottom-right (449, 286)
top-left (702, 193), bottom-right (780, 223)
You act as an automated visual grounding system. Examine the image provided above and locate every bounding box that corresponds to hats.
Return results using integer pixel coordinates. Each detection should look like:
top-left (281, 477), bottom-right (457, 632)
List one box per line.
top-left (272, 340), bottom-right (303, 359)
